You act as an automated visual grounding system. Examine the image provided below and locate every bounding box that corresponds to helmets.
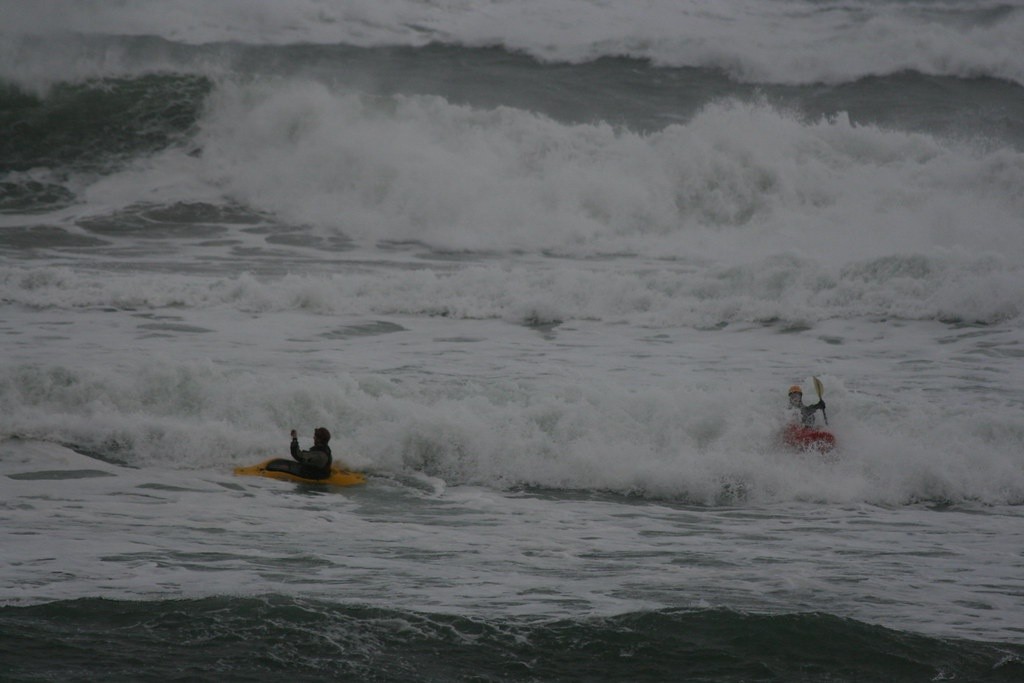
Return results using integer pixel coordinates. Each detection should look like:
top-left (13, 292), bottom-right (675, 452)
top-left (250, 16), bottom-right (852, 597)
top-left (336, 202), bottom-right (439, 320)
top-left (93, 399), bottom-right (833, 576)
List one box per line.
top-left (788, 385), bottom-right (802, 396)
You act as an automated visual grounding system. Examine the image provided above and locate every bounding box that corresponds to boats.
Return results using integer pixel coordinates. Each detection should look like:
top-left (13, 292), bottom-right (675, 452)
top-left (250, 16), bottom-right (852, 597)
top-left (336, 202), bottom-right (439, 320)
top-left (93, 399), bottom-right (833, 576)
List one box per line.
top-left (233, 457), bottom-right (368, 486)
top-left (783, 424), bottom-right (837, 455)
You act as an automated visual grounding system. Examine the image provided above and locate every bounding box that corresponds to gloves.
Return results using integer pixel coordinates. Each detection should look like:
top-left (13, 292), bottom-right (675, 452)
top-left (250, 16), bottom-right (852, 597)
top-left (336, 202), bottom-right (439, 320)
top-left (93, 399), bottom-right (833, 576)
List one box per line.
top-left (815, 401), bottom-right (825, 409)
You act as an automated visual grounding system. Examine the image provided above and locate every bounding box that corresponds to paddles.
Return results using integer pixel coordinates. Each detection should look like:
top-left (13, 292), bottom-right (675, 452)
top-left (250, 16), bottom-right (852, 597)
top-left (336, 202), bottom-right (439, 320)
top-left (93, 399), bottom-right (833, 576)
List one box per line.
top-left (812, 375), bottom-right (829, 425)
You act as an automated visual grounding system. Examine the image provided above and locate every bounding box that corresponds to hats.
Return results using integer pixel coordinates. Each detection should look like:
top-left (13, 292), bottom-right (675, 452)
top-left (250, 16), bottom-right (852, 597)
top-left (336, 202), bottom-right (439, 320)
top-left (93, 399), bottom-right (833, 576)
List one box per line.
top-left (315, 428), bottom-right (330, 443)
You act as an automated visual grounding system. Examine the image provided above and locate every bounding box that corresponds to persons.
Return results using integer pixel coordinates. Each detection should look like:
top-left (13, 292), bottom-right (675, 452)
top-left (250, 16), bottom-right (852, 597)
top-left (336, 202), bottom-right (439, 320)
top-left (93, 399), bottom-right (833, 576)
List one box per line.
top-left (289, 426), bottom-right (335, 478)
top-left (787, 382), bottom-right (827, 436)
